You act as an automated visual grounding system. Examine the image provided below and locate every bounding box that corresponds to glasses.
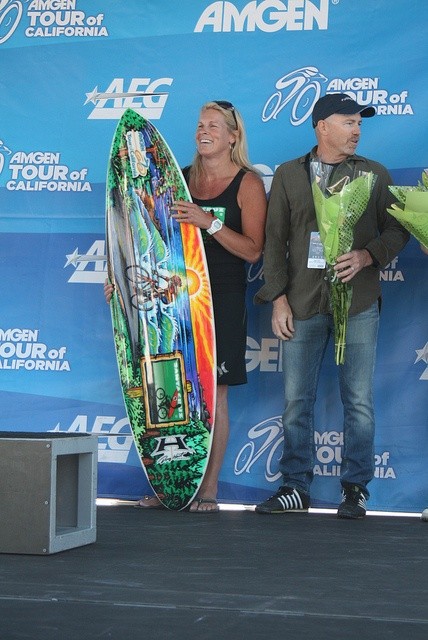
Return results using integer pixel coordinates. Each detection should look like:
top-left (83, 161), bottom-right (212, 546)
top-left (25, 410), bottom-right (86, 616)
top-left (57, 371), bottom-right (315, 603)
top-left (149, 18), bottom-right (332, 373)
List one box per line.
top-left (213, 101), bottom-right (238, 131)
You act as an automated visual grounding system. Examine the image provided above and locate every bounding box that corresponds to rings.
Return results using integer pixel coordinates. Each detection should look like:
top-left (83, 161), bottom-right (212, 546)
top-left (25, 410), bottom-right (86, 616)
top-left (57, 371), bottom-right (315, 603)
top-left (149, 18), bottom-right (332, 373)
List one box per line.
top-left (350, 267), bottom-right (355, 271)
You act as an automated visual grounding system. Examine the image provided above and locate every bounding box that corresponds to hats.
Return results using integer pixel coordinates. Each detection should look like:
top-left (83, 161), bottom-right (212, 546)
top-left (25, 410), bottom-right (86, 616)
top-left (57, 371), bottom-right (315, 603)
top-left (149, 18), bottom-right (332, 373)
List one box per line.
top-left (312, 94), bottom-right (376, 127)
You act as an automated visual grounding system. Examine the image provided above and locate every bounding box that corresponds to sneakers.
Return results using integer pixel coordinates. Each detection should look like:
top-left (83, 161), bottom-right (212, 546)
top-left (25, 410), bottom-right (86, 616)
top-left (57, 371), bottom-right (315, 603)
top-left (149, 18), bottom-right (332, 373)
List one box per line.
top-left (337, 491), bottom-right (366, 519)
top-left (255, 488), bottom-right (310, 513)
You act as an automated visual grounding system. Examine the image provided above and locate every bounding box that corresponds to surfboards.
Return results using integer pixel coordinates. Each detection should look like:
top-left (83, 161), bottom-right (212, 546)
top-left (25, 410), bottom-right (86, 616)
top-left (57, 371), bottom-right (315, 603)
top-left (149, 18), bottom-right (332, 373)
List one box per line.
top-left (105, 107), bottom-right (217, 512)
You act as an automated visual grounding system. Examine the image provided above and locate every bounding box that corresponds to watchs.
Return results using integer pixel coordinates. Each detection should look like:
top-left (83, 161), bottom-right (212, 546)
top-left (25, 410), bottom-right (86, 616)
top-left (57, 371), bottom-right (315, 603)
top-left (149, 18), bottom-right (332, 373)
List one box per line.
top-left (206, 218), bottom-right (223, 235)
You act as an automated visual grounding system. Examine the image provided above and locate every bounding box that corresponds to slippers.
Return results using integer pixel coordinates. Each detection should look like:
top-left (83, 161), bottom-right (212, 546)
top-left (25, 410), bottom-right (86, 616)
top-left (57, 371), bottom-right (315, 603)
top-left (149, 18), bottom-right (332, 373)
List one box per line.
top-left (189, 499), bottom-right (219, 514)
top-left (134, 495), bottom-right (164, 509)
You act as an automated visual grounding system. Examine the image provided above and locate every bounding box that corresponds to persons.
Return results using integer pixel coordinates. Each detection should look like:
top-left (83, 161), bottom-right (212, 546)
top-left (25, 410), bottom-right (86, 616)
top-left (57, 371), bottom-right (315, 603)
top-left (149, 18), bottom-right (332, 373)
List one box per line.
top-left (103, 101), bottom-right (267, 513)
top-left (255, 94), bottom-right (410, 519)
